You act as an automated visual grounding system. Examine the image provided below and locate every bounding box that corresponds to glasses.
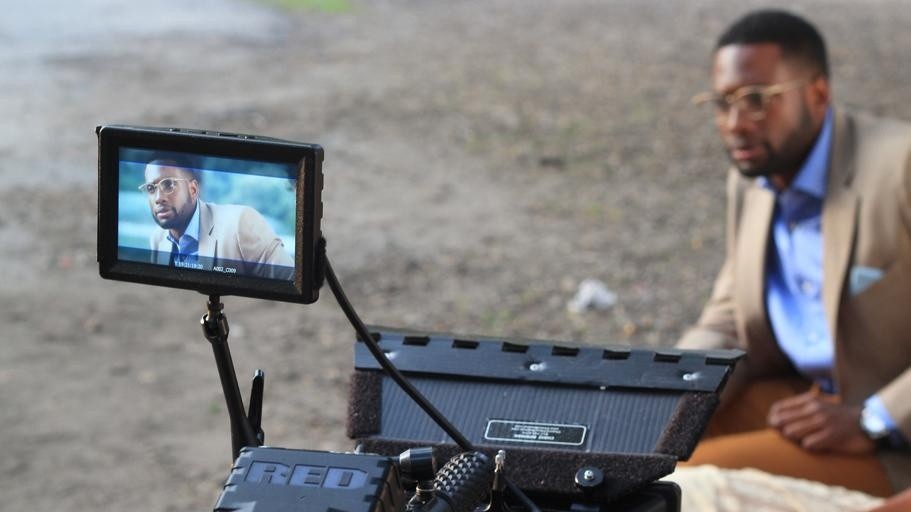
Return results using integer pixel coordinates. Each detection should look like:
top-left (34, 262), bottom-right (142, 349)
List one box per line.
top-left (692, 79), bottom-right (813, 122)
top-left (138, 178), bottom-right (190, 196)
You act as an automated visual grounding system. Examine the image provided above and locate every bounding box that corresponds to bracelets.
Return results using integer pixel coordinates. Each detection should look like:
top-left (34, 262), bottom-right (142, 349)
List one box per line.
top-left (860, 401), bottom-right (893, 448)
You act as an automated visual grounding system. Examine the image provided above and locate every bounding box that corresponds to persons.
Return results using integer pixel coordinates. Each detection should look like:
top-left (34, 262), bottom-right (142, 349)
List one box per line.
top-left (670, 9), bottom-right (911, 509)
top-left (137, 156), bottom-right (295, 284)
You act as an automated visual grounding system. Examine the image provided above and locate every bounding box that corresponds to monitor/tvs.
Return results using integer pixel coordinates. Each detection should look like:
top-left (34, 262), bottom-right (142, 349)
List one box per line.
top-left (95, 122), bottom-right (326, 305)
